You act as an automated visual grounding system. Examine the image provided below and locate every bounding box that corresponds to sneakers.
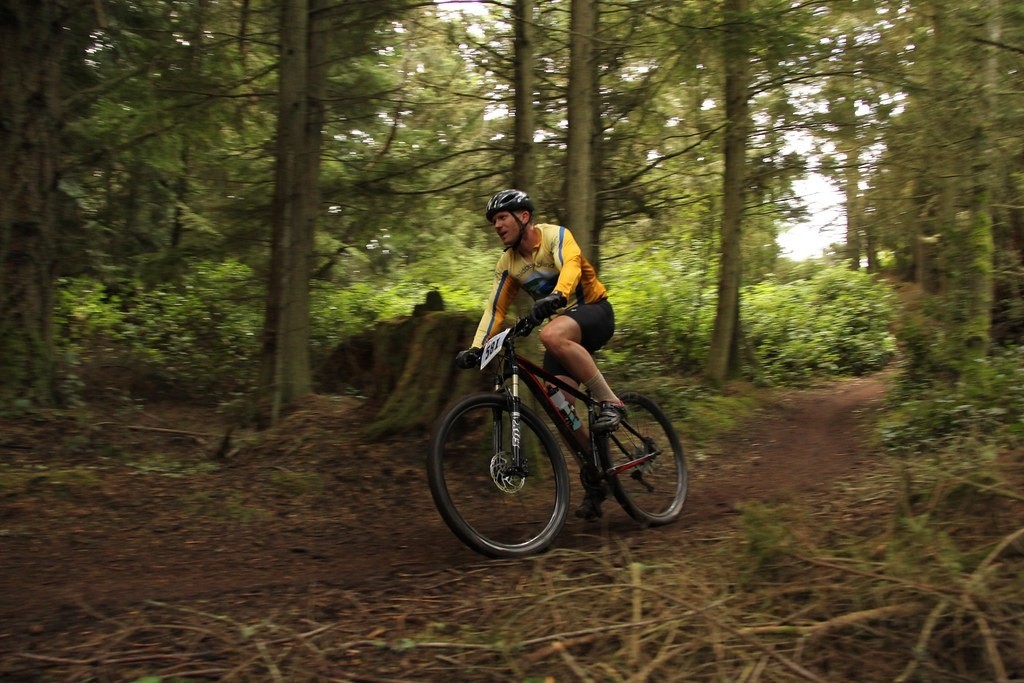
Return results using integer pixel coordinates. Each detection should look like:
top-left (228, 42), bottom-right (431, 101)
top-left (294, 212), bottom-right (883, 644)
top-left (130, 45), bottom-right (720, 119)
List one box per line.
top-left (590, 400), bottom-right (627, 433)
top-left (575, 489), bottom-right (607, 518)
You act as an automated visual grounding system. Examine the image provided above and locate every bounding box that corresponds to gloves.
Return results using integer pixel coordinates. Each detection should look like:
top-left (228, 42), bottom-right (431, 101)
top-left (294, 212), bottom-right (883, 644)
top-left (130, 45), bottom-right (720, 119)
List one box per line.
top-left (456, 346), bottom-right (484, 369)
top-left (532, 290), bottom-right (567, 319)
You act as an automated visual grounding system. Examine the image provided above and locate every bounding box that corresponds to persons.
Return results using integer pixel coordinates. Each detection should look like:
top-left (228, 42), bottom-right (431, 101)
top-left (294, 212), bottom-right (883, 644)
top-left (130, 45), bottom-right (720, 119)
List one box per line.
top-left (457, 187), bottom-right (627, 519)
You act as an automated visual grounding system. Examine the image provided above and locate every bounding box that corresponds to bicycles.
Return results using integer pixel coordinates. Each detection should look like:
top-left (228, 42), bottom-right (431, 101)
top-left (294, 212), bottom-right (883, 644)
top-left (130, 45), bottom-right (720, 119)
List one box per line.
top-left (424, 304), bottom-right (689, 560)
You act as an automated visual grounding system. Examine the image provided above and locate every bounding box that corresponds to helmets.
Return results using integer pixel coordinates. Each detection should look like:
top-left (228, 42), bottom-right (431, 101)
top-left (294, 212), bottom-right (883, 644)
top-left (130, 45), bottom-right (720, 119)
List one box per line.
top-left (486, 189), bottom-right (535, 222)
